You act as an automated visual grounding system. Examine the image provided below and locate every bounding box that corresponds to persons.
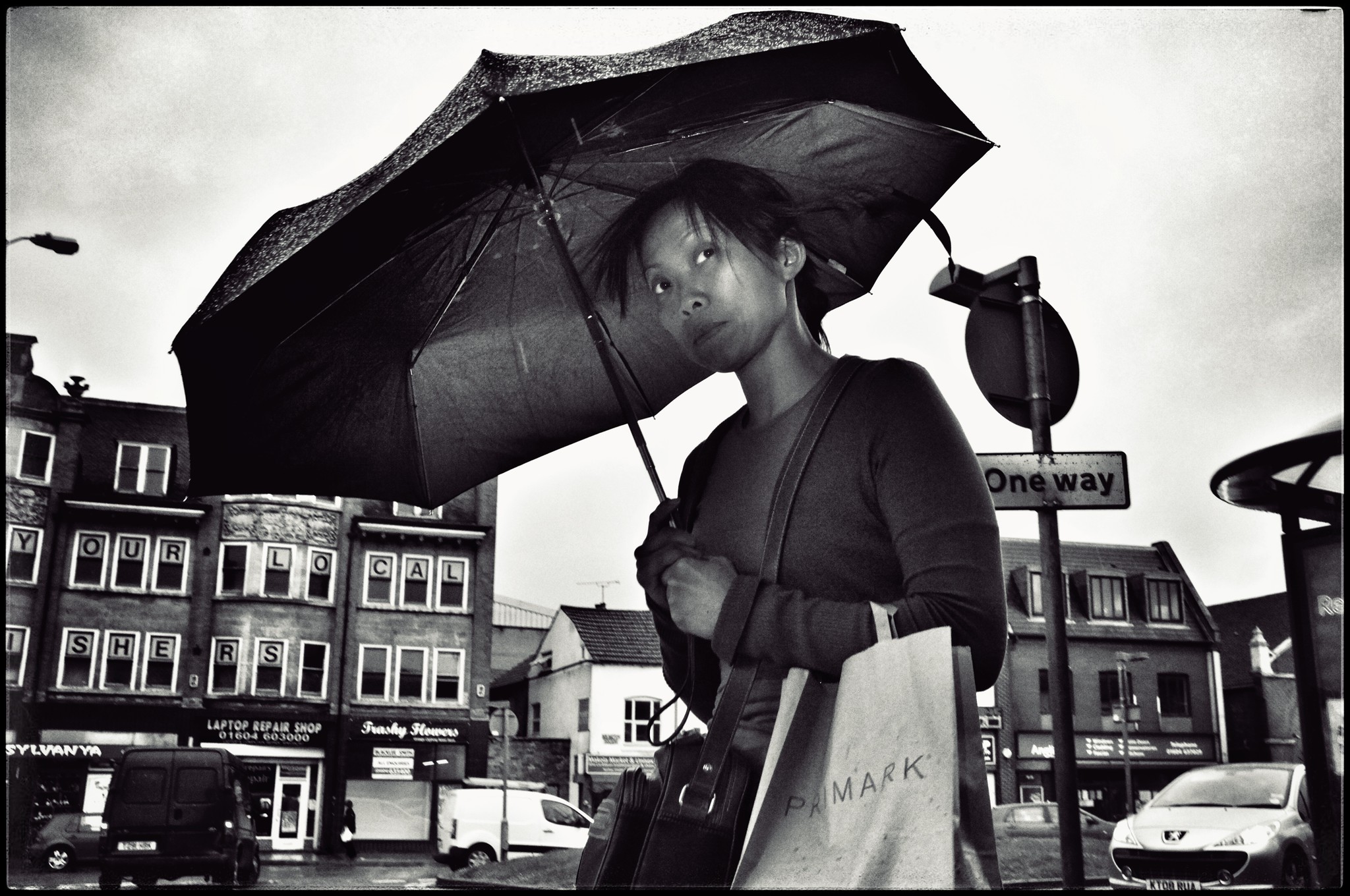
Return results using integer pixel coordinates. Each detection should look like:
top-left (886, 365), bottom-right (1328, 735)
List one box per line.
top-left (340, 801), bottom-right (356, 858)
top-left (578, 155), bottom-right (1005, 864)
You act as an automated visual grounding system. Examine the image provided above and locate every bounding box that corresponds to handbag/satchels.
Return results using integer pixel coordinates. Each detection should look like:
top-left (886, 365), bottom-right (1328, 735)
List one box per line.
top-left (575, 730), bottom-right (750, 889)
top-left (731, 603), bottom-right (1004, 893)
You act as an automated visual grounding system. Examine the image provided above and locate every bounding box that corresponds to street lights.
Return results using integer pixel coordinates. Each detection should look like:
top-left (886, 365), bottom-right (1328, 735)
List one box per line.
top-left (1113, 650), bottom-right (1149, 820)
top-left (929, 256), bottom-right (1083, 893)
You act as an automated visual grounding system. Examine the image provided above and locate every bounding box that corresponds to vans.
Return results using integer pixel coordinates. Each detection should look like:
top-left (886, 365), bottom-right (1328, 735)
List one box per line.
top-left (428, 789), bottom-right (594, 874)
top-left (100, 745), bottom-right (271, 888)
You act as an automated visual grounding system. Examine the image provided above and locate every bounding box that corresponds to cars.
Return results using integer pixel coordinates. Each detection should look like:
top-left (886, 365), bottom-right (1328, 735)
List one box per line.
top-left (988, 802), bottom-right (1117, 852)
top-left (26, 813), bottom-right (103, 872)
top-left (1108, 763), bottom-right (1317, 888)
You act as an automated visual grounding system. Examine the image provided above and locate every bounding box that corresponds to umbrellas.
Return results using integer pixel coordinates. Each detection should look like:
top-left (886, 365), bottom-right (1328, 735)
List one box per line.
top-left (168, 9), bottom-right (998, 748)
